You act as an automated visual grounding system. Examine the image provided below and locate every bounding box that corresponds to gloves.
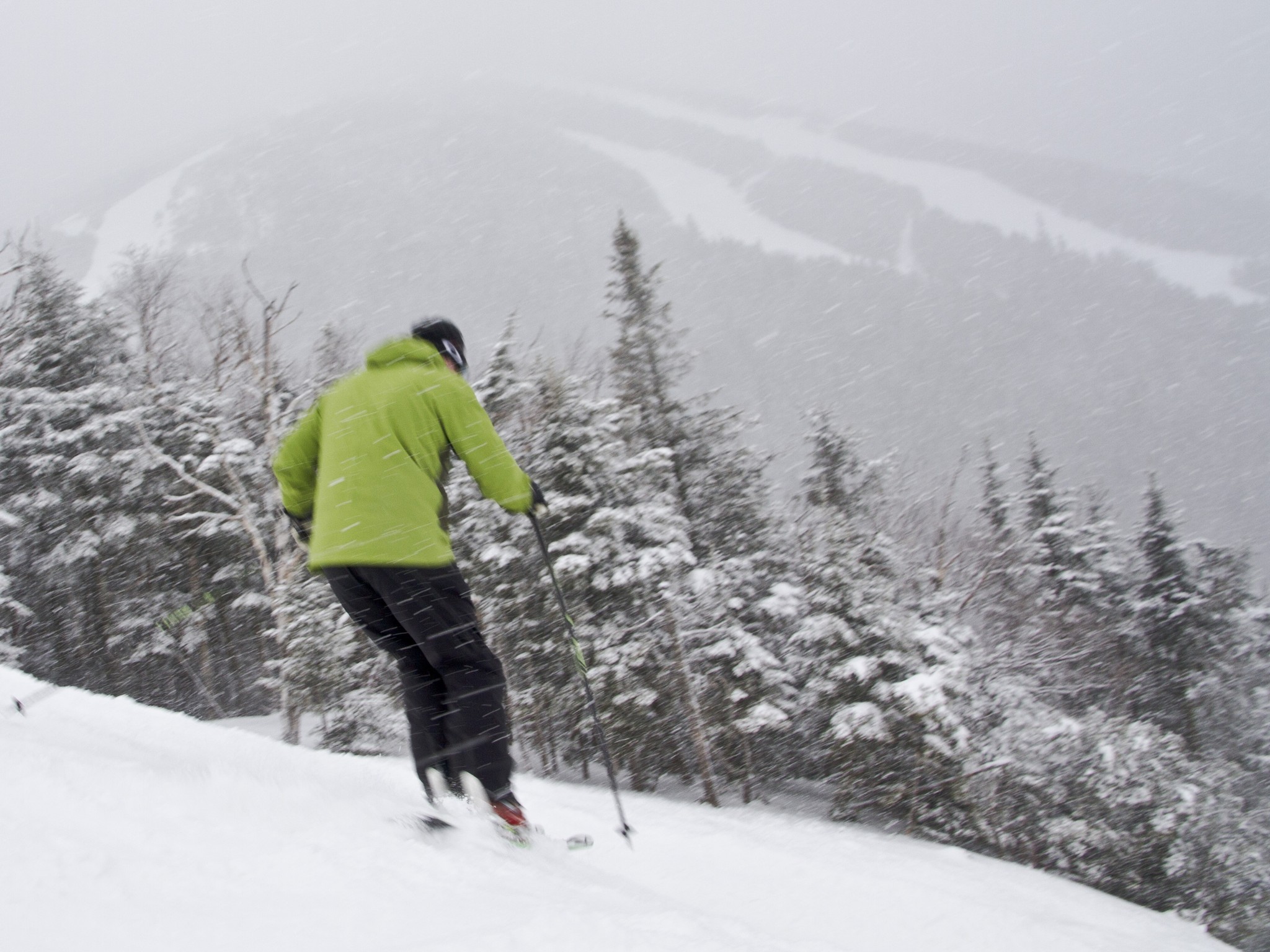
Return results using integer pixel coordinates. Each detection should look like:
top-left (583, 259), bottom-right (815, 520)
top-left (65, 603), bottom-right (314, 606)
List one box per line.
top-left (284, 508), bottom-right (313, 544)
top-left (523, 480), bottom-right (548, 514)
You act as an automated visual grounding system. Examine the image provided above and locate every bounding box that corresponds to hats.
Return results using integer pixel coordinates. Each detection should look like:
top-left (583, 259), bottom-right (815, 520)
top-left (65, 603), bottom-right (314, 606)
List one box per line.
top-left (414, 319), bottom-right (467, 370)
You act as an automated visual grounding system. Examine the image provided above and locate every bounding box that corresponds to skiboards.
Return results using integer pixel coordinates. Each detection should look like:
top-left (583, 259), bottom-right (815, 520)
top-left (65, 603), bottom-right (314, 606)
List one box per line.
top-left (416, 807), bottom-right (595, 847)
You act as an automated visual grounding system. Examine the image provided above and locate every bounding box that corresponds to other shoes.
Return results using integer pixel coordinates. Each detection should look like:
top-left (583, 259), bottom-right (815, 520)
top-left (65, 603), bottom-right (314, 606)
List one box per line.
top-left (487, 789), bottom-right (526, 830)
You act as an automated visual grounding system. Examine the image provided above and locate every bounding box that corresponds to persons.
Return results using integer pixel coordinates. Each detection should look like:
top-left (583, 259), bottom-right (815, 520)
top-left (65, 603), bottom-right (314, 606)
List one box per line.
top-left (273, 317), bottom-right (548, 831)
